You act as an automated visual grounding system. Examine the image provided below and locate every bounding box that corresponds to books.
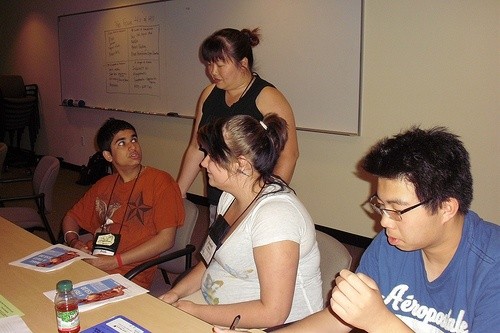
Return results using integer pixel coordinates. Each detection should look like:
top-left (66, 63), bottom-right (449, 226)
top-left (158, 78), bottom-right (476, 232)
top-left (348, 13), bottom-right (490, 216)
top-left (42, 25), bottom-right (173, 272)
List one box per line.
top-left (8, 243), bottom-right (98, 273)
top-left (78, 315), bottom-right (152, 333)
top-left (41, 272), bottom-right (150, 314)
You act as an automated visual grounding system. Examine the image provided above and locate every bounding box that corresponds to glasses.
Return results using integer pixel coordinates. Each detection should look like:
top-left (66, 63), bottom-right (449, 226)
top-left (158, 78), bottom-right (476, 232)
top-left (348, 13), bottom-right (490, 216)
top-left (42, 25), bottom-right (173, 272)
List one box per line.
top-left (367, 193), bottom-right (433, 221)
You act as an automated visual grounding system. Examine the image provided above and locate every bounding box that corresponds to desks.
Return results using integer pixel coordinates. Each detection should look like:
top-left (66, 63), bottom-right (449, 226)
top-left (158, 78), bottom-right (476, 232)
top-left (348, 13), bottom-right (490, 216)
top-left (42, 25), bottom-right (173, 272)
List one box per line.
top-left (0, 217), bottom-right (216, 333)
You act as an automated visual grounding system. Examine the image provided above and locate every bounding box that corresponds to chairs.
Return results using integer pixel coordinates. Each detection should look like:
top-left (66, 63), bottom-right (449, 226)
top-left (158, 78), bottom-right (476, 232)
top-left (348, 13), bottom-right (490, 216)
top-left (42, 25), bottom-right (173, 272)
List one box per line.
top-left (315, 230), bottom-right (352, 309)
top-left (79, 198), bottom-right (199, 298)
top-left (0, 156), bottom-right (61, 245)
top-left (0, 74), bottom-right (44, 152)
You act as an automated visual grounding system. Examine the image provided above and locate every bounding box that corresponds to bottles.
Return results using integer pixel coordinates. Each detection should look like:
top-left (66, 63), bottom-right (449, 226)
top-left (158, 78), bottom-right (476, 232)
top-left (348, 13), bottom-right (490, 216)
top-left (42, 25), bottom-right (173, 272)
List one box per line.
top-left (55, 280), bottom-right (80, 333)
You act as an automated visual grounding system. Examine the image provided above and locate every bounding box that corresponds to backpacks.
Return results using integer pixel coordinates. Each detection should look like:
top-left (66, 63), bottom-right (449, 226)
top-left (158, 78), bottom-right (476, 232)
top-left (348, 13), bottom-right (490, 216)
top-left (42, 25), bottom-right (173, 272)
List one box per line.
top-left (79, 152), bottom-right (113, 185)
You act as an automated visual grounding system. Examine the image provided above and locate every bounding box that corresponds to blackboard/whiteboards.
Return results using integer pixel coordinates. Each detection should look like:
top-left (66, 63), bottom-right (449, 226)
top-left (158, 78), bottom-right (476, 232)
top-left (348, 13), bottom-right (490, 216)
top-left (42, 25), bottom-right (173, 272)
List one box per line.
top-left (56, 1), bottom-right (364, 138)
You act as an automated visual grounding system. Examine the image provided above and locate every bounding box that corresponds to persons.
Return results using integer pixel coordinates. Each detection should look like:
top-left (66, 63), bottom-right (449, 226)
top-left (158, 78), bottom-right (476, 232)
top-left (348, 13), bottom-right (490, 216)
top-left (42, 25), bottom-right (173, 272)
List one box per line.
top-left (176, 27), bottom-right (299, 229)
top-left (157, 113), bottom-right (324, 328)
top-left (270, 123), bottom-right (500, 333)
top-left (63, 117), bottom-right (186, 290)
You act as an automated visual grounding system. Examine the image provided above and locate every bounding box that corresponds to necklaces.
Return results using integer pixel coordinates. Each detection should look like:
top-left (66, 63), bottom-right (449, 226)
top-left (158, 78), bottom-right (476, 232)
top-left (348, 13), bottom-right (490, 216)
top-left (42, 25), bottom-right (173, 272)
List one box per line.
top-left (238, 76), bottom-right (254, 98)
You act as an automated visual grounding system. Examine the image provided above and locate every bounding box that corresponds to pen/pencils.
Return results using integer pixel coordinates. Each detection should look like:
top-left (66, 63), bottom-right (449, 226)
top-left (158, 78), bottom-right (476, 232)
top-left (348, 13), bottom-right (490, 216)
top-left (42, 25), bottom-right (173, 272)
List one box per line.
top-left (230, 314), bottom-right (240, 330)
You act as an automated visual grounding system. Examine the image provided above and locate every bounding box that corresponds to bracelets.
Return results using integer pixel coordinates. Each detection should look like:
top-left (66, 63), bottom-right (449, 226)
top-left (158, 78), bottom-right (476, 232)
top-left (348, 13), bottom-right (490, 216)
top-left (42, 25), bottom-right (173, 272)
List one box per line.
top-left (115, 254), bottom-right (123, 268)
top-left (64, 230), bottom-right (79, 243)
